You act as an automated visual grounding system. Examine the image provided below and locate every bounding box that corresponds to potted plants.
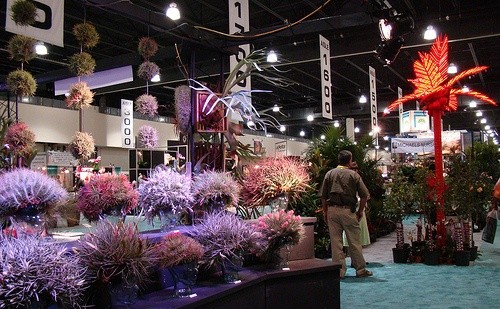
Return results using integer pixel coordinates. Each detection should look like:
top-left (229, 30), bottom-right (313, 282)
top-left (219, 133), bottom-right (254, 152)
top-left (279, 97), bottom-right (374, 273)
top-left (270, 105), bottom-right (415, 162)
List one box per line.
top-left (378, 153), bottom-right (493, 265)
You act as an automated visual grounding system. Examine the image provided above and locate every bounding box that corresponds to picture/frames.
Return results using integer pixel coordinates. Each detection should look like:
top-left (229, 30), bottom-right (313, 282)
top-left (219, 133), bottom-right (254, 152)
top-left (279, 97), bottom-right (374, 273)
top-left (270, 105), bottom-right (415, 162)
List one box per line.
top-left (194, 92), bottom-right (229, 133)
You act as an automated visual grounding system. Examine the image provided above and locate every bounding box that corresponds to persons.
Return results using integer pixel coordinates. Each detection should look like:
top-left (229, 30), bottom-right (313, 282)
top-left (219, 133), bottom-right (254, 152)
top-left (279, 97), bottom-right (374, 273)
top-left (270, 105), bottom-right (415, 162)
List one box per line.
top-left (320, 150), bottom-right (373, 278)
top-left (343, 162), bottom-right (370, 267)
top-left (492, 178), bottom-right (500, 210)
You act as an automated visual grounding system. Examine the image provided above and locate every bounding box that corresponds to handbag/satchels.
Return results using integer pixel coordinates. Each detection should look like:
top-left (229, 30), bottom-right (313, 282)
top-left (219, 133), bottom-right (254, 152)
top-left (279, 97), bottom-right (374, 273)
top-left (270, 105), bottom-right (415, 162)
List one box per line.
top-left (482, 208), bottom-right (497, 244)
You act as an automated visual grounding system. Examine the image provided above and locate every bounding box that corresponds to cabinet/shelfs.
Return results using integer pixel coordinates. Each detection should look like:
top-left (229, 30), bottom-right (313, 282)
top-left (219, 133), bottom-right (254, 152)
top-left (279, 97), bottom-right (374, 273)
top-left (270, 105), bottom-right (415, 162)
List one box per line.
top-left (56, 216), bottom-right (342, 309)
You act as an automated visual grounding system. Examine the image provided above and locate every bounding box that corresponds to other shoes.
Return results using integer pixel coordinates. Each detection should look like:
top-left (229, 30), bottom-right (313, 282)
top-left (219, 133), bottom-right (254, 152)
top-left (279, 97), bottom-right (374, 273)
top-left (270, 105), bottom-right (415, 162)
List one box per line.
top-left (356, 270), bottom-right (373, 277)
top-left (340, 272), bottom-right (345, 278)
